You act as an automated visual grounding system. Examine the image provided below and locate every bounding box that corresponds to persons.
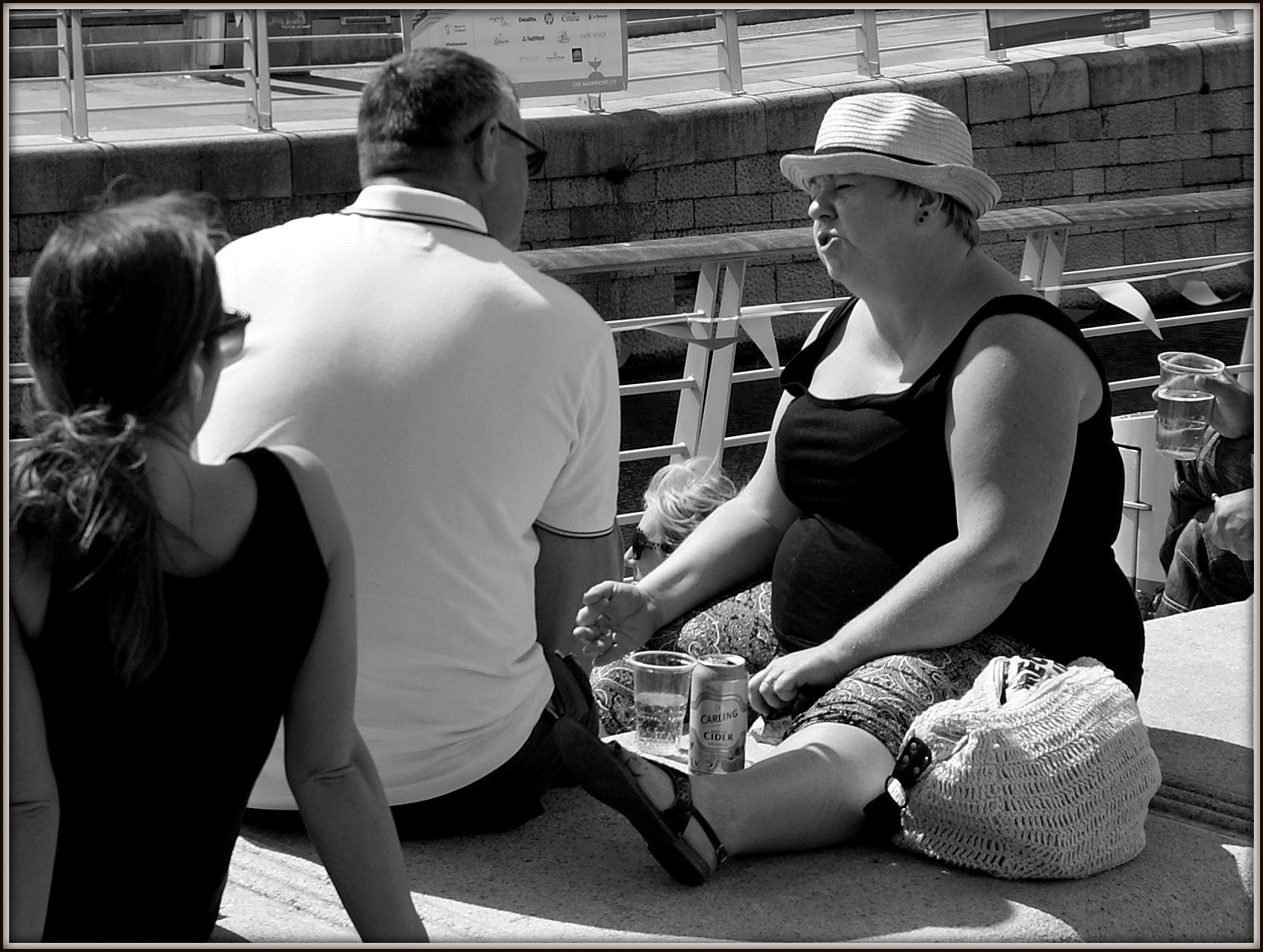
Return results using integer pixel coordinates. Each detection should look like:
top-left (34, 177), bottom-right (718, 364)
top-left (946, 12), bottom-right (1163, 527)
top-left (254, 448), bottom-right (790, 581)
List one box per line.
top-left (189, 43), bottom-right (622, 840)
top-left (8, 174), bottom-right (433, 945)
top-left (556, 89), bottom-right (1147, 888)
top-left (1144, 288), bottom-right (1254, 622)
top-left (622, 455), bottom-right (744, 588)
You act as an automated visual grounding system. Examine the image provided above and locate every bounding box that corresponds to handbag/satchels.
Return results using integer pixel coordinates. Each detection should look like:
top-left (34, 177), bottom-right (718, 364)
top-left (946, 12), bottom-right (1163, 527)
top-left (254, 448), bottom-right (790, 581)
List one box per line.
top-left (862, 652), bottom-right (1165, 881)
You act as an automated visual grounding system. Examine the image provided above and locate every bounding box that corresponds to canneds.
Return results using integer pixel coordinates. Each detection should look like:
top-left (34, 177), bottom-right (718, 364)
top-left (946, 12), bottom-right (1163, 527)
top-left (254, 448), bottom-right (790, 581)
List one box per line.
top-left (685, 647), bottom-right (750, 779)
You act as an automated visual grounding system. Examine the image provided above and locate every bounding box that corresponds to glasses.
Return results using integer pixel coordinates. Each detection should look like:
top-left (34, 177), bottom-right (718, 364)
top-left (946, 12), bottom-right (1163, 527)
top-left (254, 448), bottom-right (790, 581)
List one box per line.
top-left (632, 526), bottom-right (674, 561)
top-left (203, 307), bottom-right (251, 358)
top-left (463, 119), bottom-right (549, 178)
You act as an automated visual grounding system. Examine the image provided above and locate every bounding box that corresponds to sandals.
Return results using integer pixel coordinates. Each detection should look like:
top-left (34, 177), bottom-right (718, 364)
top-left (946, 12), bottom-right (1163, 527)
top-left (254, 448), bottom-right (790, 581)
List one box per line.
top-left (554, 714), bottom-right (730, 888)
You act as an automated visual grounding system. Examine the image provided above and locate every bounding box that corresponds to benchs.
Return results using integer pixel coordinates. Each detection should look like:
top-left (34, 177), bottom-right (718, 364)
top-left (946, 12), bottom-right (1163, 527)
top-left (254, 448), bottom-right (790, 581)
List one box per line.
top-left (199, 591), bottom-right (1254, 942)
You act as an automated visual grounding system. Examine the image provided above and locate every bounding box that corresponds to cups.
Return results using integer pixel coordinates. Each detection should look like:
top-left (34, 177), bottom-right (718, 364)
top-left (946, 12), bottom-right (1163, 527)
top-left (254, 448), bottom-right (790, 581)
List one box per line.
top-left (628, 650), bottom-right (697, 756)
top-left (1154, 352), bottom-right (1225, 459)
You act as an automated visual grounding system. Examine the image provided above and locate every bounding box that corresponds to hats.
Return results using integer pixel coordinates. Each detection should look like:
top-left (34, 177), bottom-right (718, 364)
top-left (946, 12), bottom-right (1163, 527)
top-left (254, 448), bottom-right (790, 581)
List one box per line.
top-left (780, 91), bottom-right (1003, 220)
